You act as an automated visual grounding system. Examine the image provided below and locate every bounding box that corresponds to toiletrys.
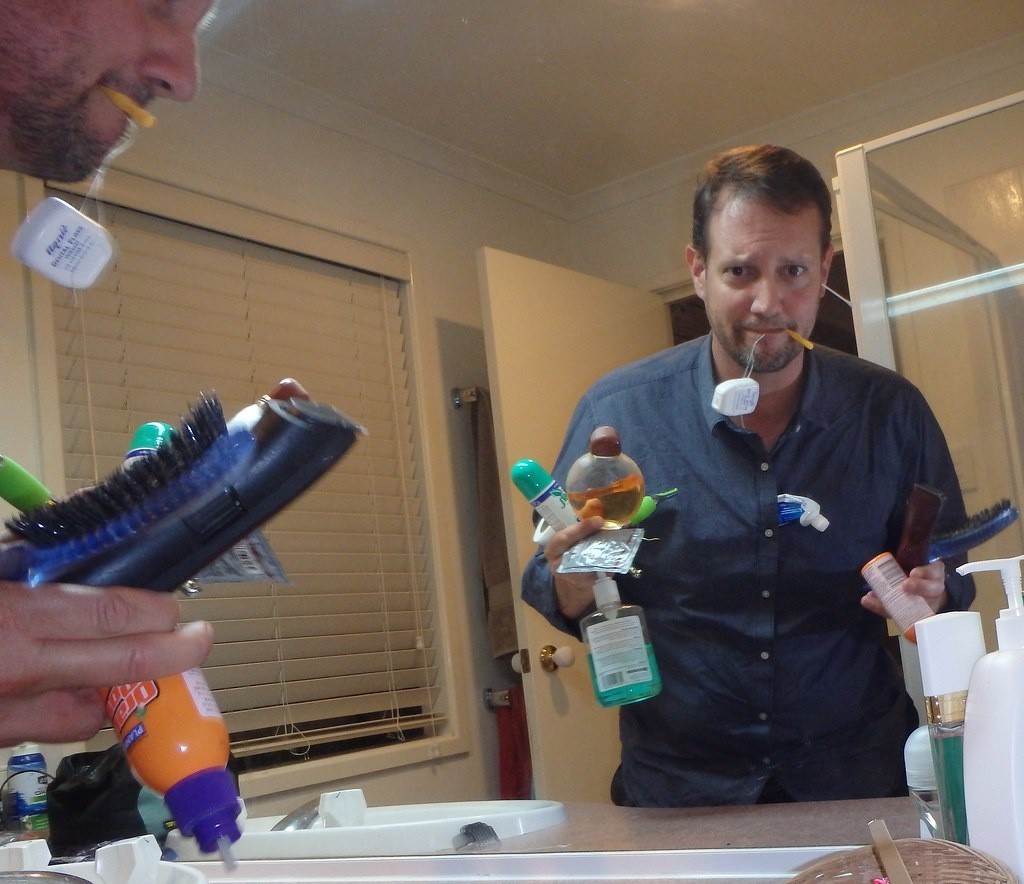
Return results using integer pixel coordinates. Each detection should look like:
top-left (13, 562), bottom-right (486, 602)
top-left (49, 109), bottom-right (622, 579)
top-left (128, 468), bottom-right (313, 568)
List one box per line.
top-left (862, 553), bottom-right (935, 646)
top-left (956, 554), bottom-right (1024, 883)
top-left (8, 742), bottom-right (50, 813)
top-left (774, 494), bottom-right (830, 532)
top-left (89, 664), bottom-right (248, 873)
top-left (580, 571), bottom-right (662, 709)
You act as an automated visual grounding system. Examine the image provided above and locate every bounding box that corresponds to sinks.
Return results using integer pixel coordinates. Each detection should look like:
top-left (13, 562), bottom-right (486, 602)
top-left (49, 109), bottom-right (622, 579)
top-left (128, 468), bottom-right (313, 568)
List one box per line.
top-left (164, 792), bottom-right (568, 862)
top-left (0, 853), bottom-right (205, 883)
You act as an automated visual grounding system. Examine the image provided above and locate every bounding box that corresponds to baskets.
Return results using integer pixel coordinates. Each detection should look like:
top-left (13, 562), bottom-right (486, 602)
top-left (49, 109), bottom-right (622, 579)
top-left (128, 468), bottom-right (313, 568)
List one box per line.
top-left (787, 819), bottom-right (1018, 884)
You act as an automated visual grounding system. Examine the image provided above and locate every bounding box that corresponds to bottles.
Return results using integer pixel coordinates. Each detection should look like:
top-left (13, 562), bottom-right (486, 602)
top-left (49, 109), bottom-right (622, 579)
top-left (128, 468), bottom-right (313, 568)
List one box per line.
top-left (861, 551), bottom-right (935, 645)
top-left (912, 612), bottom-right (986, 850)
top-left (905, 724), bottom-right (943, 841)
top-left (122, 420), bottom-right (179, 471)
top-left (509, 458), bottom-right (582, 532)
top-left (221, 377), bottom-right (310, 437)
top-left (94, 665), bottom-right (244, 874)
top-left (566, 426), bottom-right (645, 529)
top-left (6, 742), bottom-right (52, 841)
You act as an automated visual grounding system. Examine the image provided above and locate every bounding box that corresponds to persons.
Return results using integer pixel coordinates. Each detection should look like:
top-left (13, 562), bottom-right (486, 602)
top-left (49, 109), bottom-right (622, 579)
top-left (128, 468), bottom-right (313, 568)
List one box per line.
top-left (517, 143), bottom-right (981, 815)
top-left (0, 0), bottom-right (219, 751)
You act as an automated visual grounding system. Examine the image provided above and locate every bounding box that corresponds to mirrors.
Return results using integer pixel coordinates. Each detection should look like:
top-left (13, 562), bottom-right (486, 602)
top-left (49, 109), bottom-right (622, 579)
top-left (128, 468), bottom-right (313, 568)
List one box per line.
top-left (2, 0), bottom-right (1024, 874)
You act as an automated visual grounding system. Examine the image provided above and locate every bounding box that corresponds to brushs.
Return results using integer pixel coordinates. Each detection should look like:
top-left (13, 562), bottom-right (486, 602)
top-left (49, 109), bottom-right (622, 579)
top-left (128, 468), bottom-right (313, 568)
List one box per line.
top-left (923, 498), bottom-right (1017, 563)
top-left (1, 383), bottom-right (257, 597)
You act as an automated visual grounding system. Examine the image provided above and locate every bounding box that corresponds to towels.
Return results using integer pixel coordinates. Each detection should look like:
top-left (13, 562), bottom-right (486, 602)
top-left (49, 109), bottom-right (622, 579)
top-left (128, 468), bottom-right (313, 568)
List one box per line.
top-left (497, 686), bottom-right (533, 800)
top-left (467, 387), bottom-right (518, 662)
